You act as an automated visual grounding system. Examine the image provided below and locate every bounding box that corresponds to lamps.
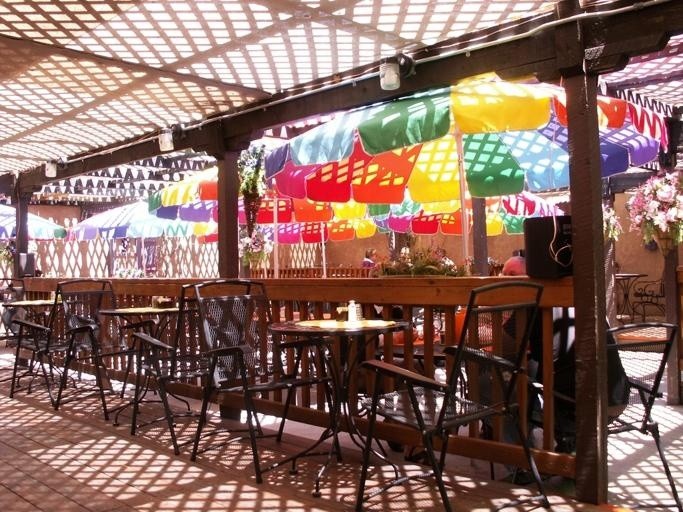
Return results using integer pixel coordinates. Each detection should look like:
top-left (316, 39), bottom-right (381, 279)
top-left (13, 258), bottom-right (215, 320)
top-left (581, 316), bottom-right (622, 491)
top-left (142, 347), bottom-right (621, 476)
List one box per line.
top-left (42, 158), bottom-right (65, 179)
top-left (155, 123), bottom-right (186, 153)
top-left (375, 52), bottom-right (418, 91)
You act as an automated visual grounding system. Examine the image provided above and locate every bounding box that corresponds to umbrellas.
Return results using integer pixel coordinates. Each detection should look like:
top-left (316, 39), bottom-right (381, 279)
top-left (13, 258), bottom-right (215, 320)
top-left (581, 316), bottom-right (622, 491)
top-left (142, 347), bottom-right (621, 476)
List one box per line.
top-left (263, 82), bottom-right (668, 277)
top-left (0, 203), bottom-right (67, 241)
top-left (67, 199), bottom-right (217, 278)
top-left (262, 216), bottom-right (392, 279)
top-left (367, 186), bottom-right (566, 236)
top-left (147, 166), bottom-right (422, 280)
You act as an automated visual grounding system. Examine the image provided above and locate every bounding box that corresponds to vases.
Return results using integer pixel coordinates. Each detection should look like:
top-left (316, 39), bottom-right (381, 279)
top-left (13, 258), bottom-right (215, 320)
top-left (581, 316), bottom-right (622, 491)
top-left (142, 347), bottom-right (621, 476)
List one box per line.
top-left (243, 192), bottom-right (263, 236)
top-left (7, 260), bottom-right (15, 278)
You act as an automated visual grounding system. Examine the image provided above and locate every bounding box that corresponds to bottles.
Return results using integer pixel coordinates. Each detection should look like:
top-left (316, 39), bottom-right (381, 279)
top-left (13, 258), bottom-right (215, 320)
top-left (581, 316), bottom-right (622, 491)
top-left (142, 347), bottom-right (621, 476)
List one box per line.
top-left (347, 300), bottom-right (357, 325)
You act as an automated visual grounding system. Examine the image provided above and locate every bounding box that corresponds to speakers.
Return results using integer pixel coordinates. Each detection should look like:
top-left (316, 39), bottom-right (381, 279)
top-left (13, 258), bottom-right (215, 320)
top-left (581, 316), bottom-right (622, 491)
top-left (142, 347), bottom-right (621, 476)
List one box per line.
top-left (524, 215), bottom-right (569, 279)
top-left (17, 251), bottom-right (35, 277)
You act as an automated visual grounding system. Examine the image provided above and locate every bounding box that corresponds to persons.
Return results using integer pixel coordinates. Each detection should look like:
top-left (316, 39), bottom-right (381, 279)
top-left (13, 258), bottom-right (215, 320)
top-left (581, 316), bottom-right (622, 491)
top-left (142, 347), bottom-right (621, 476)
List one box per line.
top-left (435, 248), bottom-right (457, 274)
top-left (393, 247), bottom-right (414, 270)
top-left (498, 307), bottom-right (630, 486)
top-left (502, 249), bottom-right (526, 276)
top-left (361, 248), bottom-right (377, 277)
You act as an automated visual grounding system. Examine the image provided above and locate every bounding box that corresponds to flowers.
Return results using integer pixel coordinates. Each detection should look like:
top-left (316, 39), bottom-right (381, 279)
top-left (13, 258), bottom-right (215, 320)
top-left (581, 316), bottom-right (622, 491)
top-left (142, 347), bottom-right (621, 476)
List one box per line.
top-left (236, 222), bottom-right (277, 271)
top-left (233, 144), bottom-right (279, 198)
top-left (366, 228), bottom-right (469, 277)
top-left (0, 235), bottom-right (20, 264)
top-left (600, 200), bottom-right (623, 250)
top-left (620, 168), bottom-right (683, 258)
